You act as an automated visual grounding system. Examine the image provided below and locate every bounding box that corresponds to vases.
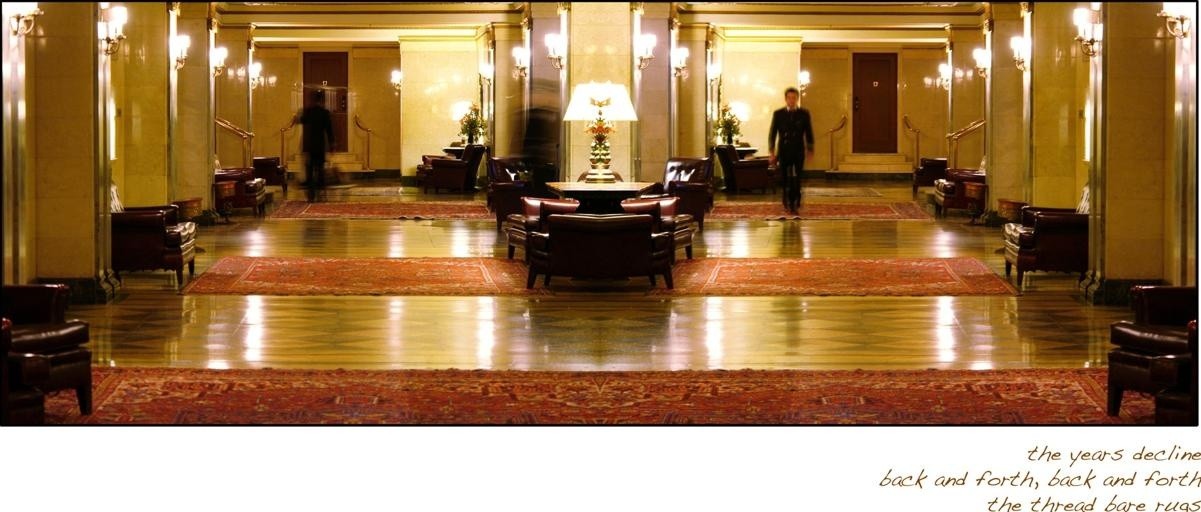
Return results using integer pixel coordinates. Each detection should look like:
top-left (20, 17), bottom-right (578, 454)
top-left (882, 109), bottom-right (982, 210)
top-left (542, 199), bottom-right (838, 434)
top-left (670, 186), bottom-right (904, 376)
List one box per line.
top-left (468, 134), bottom-right (473, 143)
top-left (727, 135), bottom-right (733, 145)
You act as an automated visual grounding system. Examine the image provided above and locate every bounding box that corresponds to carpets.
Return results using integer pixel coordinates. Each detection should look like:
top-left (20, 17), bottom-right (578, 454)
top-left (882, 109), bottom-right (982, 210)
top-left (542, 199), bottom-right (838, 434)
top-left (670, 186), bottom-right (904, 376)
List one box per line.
top-left (265, 200), bottom-right (932, 221)
top-left (179, 255), bottom-right (1021, 296)
top-left (0, 367), bottom-right (1156, 425)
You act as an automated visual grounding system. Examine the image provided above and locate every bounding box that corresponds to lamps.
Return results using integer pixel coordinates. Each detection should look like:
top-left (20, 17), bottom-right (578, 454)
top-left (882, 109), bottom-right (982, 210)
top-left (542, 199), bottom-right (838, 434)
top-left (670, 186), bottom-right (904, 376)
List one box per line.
top-left (3, 2), bottom-right (262, 90)
top-left (640, 34), bottom-right (658, 70)
top-left (544, 34), bottom-right (562, 70)
top-left (938, 1), bottom-right (1197, 90)
top-left (563, 80), bottom-right (640, 183)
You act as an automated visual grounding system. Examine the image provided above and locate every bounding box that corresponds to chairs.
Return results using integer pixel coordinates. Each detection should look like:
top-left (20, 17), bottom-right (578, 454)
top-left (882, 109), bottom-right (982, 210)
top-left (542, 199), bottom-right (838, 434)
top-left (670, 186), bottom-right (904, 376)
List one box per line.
top-left (913, 157), bottom-right (1197, 425)
top-left (432, 145), bottom-right (485, 194)
top-left (716, 145), bottom-right (768, 193)
top-left (1, 157), bottom-right (287, 425)
top-left (488, 156), bottom-right (714, 260)
top-left (418, 154), bottom-right (452, 193)
top-left (754, 156), bottom-right (778, 194)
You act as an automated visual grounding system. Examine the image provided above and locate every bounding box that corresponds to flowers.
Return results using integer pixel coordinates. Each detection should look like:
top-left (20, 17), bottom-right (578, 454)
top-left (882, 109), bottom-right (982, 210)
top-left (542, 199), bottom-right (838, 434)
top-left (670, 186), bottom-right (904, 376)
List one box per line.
top-left (457, 105), bottom-right (488, 136)
top-left (716, 105), bottom-right (743, 143)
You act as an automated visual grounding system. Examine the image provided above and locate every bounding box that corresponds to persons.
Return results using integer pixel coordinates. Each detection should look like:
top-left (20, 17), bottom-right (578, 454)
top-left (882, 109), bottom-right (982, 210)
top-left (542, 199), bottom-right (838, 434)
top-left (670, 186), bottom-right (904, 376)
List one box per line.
top-left (292, 90), bottom-right (335, 203)
top-left (768, 88), bottom-right (814, 216)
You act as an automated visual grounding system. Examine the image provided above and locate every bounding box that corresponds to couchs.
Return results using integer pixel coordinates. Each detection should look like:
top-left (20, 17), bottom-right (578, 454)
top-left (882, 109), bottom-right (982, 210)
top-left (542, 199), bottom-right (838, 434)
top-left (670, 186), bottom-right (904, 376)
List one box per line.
top-left (526, 212), bottom-right (674, 289)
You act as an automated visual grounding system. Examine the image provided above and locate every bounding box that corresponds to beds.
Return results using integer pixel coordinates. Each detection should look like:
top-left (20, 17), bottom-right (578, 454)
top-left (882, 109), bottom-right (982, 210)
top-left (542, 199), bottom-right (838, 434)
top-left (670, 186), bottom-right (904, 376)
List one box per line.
top-left (712, 148), bottom-right (758, 192)
